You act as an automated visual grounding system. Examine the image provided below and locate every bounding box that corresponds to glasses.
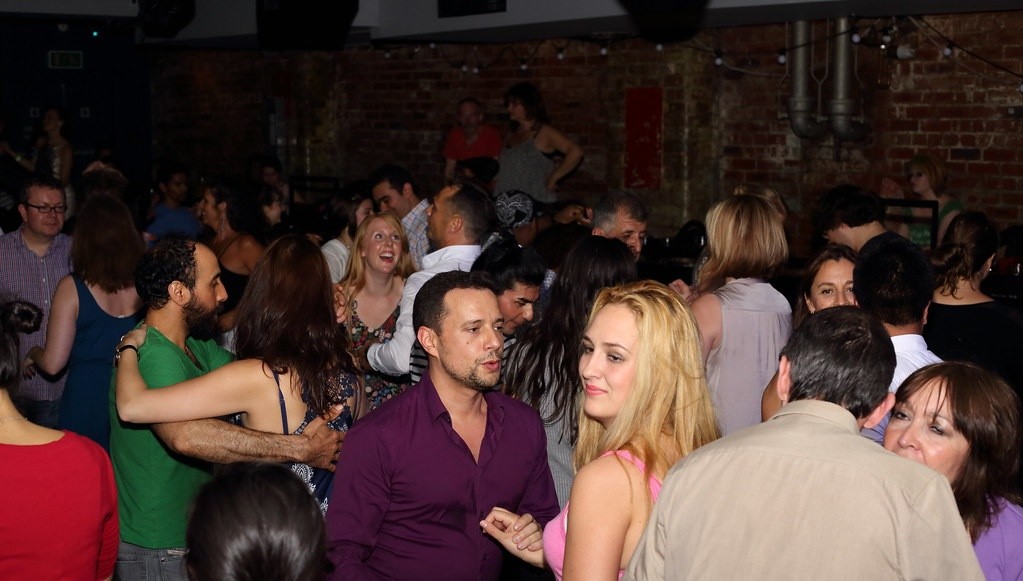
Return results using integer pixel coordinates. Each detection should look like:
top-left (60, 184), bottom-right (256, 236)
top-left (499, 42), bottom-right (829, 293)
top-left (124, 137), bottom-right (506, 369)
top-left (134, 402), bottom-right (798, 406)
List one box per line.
top-left (22, 200), bottom-right (68, 214)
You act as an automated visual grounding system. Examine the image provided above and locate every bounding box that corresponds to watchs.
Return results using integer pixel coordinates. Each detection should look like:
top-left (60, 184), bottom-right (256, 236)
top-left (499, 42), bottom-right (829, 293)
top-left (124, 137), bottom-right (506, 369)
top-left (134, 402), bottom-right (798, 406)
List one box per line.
top-left (114, 345), bottom-right (139, 366)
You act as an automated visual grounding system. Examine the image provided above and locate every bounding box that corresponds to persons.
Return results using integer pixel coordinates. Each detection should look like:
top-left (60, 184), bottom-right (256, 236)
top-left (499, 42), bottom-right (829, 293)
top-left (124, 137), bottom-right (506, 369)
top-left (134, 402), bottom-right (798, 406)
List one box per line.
top-left (479, 280), bottom-right (722, 581)
top-left (323, 269), bottom-right (561, 581)
top-left (0, 78), bottom-right (1023, 581)
